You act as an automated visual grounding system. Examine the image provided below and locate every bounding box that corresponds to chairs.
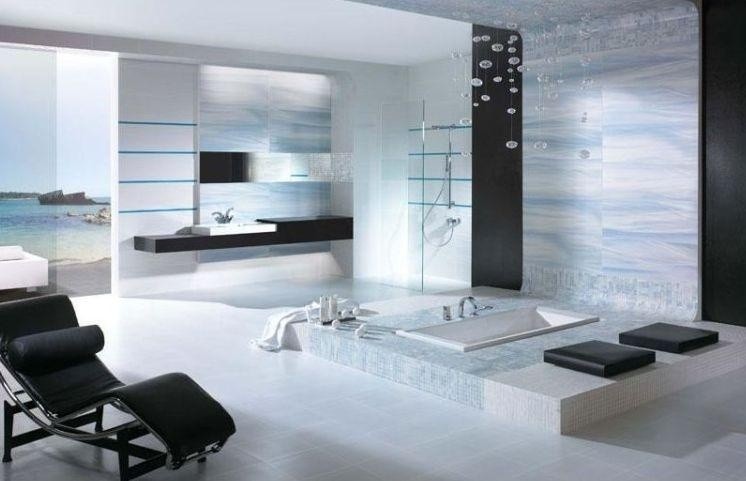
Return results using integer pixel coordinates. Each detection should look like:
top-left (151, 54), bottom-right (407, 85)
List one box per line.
top-left (0, 293), bottom-right (236, 481)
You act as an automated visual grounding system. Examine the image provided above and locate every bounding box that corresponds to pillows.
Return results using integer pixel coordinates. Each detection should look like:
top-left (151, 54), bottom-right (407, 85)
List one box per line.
top-left (9, 324), bottom-right (104, 367)
top-left (1, 245), bottom-right (23, 260)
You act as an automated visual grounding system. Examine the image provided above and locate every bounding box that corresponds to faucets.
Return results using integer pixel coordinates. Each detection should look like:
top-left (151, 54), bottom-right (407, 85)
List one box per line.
top-left (458, 295), bottom-right (479, 317)
top-left (223, 207), bottom-right (234, 222)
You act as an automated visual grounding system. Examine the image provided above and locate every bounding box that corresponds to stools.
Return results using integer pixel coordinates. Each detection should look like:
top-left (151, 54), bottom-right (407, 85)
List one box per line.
top-left (0, 251), bottom-right (49, 292)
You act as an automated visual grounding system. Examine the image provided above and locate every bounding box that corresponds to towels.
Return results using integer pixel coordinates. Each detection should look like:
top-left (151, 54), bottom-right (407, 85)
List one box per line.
top-left (249, 298), bottom-right (358, 352)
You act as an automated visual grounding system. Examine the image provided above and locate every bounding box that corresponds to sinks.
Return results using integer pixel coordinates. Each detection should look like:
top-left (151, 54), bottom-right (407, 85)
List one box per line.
top-left (191, 223), bottom-right (277, 238)
top-left (0, 244), bottom-right (49, 292)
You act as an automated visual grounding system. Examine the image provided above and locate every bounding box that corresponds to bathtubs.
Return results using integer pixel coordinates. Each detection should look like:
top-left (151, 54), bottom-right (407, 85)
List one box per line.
top-left (395, 304), bottom-right (601, 352)
